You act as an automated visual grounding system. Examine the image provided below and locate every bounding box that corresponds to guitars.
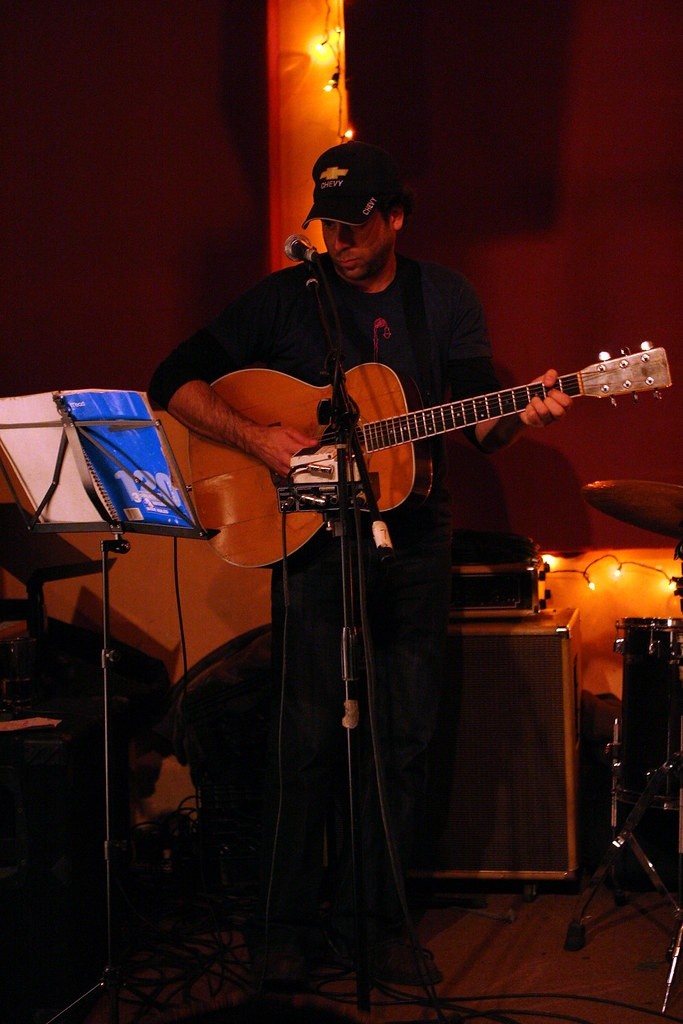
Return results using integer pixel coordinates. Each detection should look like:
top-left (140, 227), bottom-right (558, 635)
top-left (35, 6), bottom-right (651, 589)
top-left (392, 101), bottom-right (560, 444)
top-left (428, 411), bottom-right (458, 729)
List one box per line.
top-left (187, 339), bottom-right (675, 568)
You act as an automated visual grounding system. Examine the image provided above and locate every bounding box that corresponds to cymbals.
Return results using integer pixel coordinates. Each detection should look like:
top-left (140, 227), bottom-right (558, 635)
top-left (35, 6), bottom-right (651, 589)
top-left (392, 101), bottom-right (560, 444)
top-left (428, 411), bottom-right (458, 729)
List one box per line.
top-left (580, 477), bottom-right (683, 536)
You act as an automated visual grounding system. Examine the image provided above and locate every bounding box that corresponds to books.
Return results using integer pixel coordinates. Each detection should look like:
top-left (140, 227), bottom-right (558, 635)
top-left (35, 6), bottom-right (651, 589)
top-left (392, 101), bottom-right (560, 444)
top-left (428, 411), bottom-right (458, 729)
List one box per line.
top-left (0, 388), bottom-right (197, 530)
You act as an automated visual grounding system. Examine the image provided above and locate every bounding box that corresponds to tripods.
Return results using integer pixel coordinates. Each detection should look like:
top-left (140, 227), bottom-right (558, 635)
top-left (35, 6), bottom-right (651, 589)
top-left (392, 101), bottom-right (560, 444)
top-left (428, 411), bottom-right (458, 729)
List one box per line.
top-left (46, 539), bottom-right (180, 1024)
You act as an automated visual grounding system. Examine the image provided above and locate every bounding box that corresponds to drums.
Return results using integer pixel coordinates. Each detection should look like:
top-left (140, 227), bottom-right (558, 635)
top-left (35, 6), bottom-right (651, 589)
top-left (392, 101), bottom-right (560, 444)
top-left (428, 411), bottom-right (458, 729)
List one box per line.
top-left (611, 617), bottom-right (682, 810)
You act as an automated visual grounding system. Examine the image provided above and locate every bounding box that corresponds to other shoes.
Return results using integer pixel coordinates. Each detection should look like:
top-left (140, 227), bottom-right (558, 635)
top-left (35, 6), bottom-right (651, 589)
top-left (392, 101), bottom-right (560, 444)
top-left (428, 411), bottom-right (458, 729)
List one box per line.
top-left (350, 931), bottom-right (442, 986)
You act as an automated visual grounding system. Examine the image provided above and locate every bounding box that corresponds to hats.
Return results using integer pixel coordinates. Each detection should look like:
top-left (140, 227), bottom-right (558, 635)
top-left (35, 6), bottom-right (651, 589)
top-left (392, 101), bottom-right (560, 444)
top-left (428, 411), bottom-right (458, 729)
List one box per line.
top-left (301, 139), bottom-right (402, 230)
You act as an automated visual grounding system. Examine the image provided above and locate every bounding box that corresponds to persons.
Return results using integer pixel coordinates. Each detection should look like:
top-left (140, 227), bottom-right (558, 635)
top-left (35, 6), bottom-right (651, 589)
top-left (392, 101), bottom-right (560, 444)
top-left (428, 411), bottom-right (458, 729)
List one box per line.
top-left (147, 141), bottom-right (572, 986)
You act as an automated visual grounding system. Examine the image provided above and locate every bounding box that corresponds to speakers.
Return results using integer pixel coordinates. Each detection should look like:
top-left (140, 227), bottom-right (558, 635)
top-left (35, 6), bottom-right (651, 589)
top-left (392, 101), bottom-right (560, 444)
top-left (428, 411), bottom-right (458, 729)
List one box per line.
top-left (402, 608), bottom-right (581, 882)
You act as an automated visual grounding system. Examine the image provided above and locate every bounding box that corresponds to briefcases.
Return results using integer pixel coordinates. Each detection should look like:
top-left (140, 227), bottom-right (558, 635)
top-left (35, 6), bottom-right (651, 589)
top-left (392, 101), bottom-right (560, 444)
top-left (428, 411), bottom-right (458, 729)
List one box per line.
top-left (449, 560), bottom-right (551, 619)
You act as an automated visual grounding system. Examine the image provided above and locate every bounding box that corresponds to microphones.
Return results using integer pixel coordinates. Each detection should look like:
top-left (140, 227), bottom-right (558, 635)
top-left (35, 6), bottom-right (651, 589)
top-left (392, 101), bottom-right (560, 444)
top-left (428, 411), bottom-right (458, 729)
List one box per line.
top-left (281, 231), bottom-right (321, 269)
